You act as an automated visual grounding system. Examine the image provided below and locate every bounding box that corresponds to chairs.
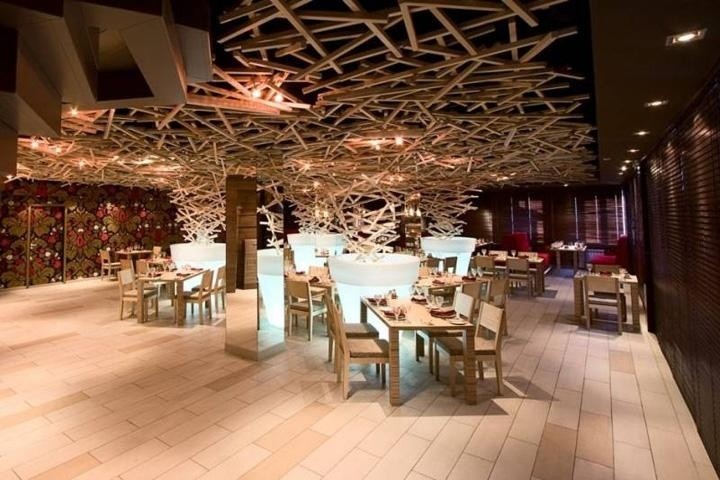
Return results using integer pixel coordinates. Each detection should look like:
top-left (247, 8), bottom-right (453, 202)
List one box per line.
top-left (175, 269), bottom-right (214, 325)
top-left (153, 246), bottom-right (162, 255)
top-left (192, 267), bottom-right (225, 314)
top-left (283, 248), bottom-right (295, 277)
top-left (585, 274), bottom-right (623, 336)
top-left (506, 257), bottom-right (533, 298)
top-left (101, 251), bottom-right (120, 280)
top-left (445, 257), bottom-right (456, 273)
top-left (308, 266), bottom-right (329, 276)
top-left (461, 283), bottom-right (480, 311)
top-left (136, 259), bottom-right (147, 273)
top-left (490, 251), bottom-right (507, 257)
top-left (476, 256), bottom-right (495, 280)
top-left (518, 252), bottom-right (538, 259)
top-left (415, 293), bottom-right (476, 374)
top-left (332, 308), bottom-right (390, 401)
top-left (328, 298), bottom-right (379, 363)
top-left (595, 266), bottom-right (627, 323)
top-left (483, 278), bottom-right (507, 335)
top-left (120, 260), bottom-right (134, 272)
top-left (117, 268), bottom-right (158, 321)
top-left (287, 280), bottom-right (327, 341)
top-left (436, 302), bottom-right (507, 397)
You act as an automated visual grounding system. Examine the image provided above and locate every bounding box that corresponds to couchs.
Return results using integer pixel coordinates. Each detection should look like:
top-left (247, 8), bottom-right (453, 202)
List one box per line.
top-left (512, 231), bottom-right (551, 267)
top-left (591, 236), bottom-right (629, 268)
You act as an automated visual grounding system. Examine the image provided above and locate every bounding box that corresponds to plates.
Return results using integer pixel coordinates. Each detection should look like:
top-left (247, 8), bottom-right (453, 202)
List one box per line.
top-left (384, 311), bottom-right (406, 319)
top-left (431, 308), bottom-right (456, 318)
top-left (367, 297), bottom-right (386, 304)
top-left (411, 297), bottom-right (426, 304)
top-left (431, 282), bottom-right (445, 286)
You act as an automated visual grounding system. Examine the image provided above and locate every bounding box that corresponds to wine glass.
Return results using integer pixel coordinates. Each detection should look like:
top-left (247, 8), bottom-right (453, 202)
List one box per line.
top-left (436, 297), bottom-right (444, 307)
top-left (482, 249), bottom-right (486, 256)
top-left (374, 294), bottom-right (382, 307)
top-left (478, 269), bottom-right (482, 278)
top-left (427, 294), bottom-right (434, 307)
top-left (587, 263), bottom-right (593, 273)
top-left (393, 307), bottom-right (401, 321)
top-left (432, 267), bottom-right (437, 277)
top-left (471, 268), bottom-right (477, 278)
top-left (511, 250), bottom-right (516, 257)
